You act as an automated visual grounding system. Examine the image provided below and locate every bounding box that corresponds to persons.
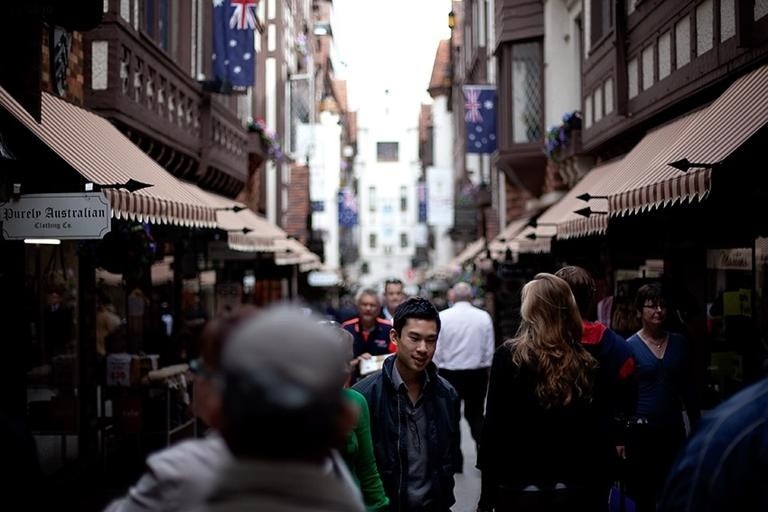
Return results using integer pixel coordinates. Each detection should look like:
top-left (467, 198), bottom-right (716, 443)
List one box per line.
top-left (340, 278), bottom-right (495, 474)
top-left (100, 298), bottom-right (464, 511)
top-left (475, 265), bottom-right (768, 511)
top-left (38, 287), bottom-right (209, 373)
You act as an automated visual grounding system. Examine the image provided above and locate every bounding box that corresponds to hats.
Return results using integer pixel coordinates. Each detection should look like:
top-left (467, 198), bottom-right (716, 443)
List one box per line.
top-left (219, 299), bottom-right (353, 412)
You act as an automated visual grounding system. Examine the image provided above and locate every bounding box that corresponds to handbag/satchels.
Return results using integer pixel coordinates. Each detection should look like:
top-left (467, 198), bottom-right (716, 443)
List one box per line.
top-left (608, 480), bottom-right (637, 510)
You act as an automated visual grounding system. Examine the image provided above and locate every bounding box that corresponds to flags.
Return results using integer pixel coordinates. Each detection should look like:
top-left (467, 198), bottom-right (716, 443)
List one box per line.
top-left (211, 0), bottom-right (258, 86)
top-left (338, 185), bottom-right (359, 227)
top-left (418, 185), bottom-right (426, 223)
top-left (462, 84), bottom-right (497, 154)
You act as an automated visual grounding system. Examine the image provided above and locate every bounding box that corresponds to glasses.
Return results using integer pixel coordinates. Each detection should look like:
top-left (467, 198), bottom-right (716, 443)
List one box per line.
top-left (642, 302), bottom-right (668, 311)
